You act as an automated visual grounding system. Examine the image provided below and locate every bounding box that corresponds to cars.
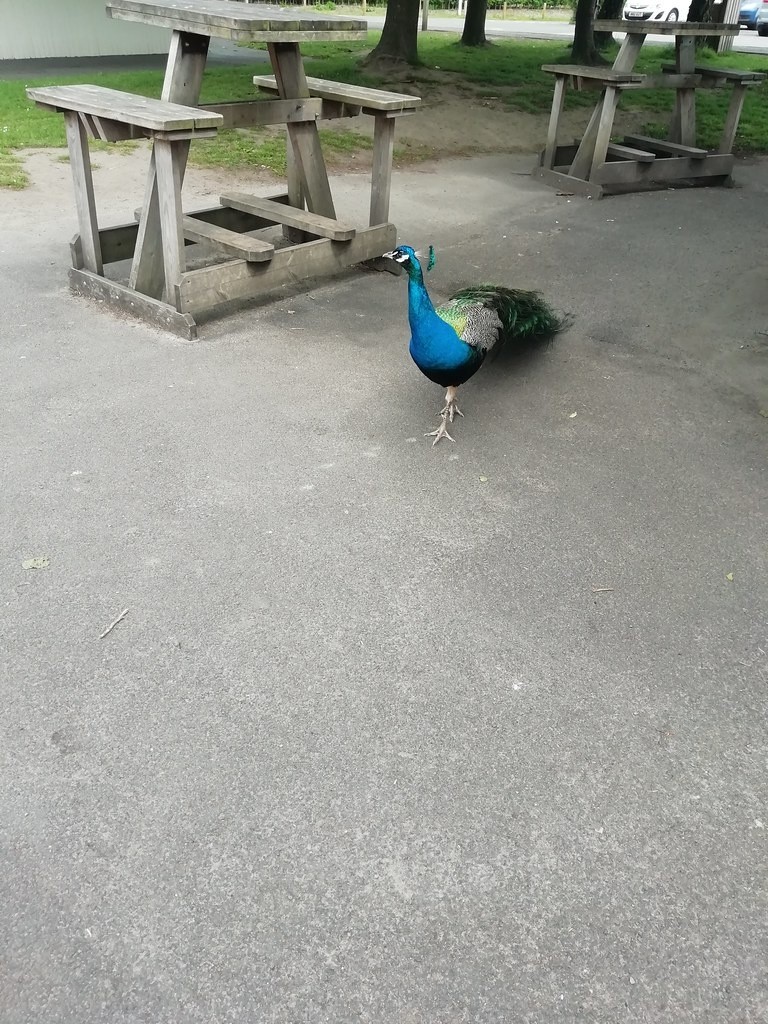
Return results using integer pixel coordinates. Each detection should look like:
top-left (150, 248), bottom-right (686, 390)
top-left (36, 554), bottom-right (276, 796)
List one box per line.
top-left (738, 0), bottom-right (768, 37)
top-left (623, 0), bottom-right (693, 22)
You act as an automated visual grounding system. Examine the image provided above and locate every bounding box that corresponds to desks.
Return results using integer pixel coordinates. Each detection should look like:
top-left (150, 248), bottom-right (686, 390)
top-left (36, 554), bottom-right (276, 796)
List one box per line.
top-left (105, 0), bottom-right (368, 300)
top-left (569, 20), bottom-right (741, 182)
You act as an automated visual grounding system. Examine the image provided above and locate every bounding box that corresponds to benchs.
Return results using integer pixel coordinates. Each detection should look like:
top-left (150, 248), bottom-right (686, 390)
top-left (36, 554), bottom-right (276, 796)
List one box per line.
top-left (662, 63), bottom-right (767, 154)
top-left (541, 65), bottom-right (647, 172)
top-left (26, 84), bottom-right (223, 303)
top-left (254, 74), bottom-right (421, 226)
